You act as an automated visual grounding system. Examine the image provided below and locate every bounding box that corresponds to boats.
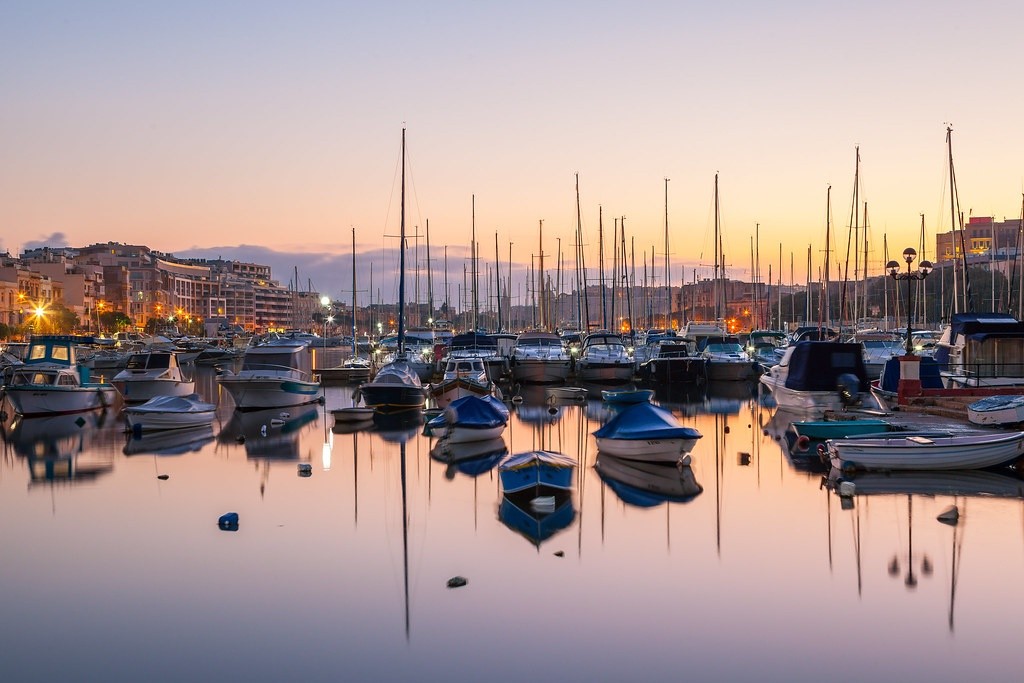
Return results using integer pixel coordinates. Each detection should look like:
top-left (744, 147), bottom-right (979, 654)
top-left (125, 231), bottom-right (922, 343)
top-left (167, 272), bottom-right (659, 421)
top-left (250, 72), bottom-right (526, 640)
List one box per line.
top-left (820, 460), bottom-right (1024, 505)
top-left (427, 393), bottom-right (510, 443)
top-left (600, 382), bottom-right (654, 404)
top-left (499, 449), bottom-right (576, 495)
top-left (122, 421), bottom-right (214, 458)
top-left (497, 489), bottom-right (578, 553)
top-left (332, 420), bottom-right (375, 434)
top-left (71, 331), bottom-right (237, 369)
top-left (218, 398), bottom-right (320, 463)
top-left (593, 447), bottom-right (705, 507)
top-left (590, 404), bottom-right (703, 456)
top-left (426, 356), bottom-right (503, 412)
top-left (784, 413), bottom-right (1024, 470)
top-left (4, 406), bottom-right (114, 481)
top-left (331, 407), bottom-right (376, 421)
top-left (431, 436), bottom-right (507, 475)
top-left (0, 335), bottom-right (322, 428)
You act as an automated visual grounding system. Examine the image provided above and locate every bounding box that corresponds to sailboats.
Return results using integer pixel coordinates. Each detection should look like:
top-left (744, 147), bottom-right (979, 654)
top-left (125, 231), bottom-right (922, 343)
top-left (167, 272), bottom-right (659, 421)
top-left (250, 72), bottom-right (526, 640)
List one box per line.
top-left (339, 121), bottom-right (1024, 412)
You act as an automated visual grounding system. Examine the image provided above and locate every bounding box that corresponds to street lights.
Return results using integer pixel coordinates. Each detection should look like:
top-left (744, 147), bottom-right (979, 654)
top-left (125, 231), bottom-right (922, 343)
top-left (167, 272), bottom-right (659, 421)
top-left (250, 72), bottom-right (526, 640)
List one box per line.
top-left (886, 248), bottom-right (933, 356)
top-left (97, 304), bottom-right (104, 335)
